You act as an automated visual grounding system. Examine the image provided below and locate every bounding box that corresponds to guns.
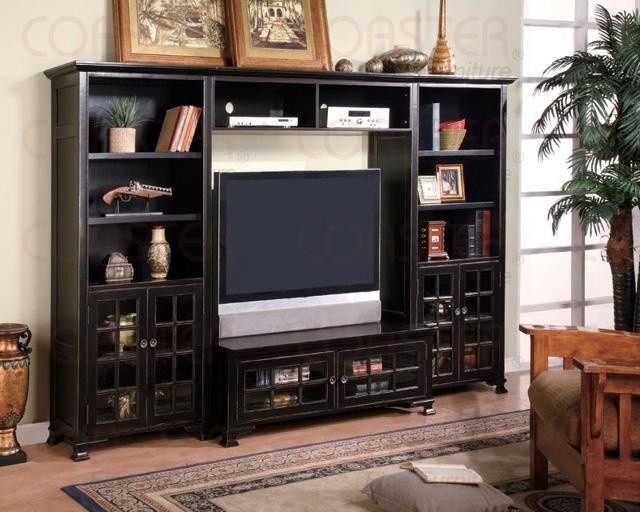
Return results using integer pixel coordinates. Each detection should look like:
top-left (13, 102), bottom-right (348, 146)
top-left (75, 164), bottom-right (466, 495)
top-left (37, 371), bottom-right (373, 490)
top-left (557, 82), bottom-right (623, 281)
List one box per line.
top-left (102, 180), bottom-right (173, 205)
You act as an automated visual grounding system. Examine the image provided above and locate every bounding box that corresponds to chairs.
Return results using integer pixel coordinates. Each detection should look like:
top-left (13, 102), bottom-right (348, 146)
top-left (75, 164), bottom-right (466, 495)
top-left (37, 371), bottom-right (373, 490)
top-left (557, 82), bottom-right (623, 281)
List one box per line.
top-left (516, 322), bottom-right (639, 511)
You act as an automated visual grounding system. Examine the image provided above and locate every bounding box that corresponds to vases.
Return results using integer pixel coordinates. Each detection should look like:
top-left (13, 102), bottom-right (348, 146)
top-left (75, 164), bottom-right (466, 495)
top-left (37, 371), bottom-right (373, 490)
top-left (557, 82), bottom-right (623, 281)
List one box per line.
top-left (0, 317), bottom-right (33, 468)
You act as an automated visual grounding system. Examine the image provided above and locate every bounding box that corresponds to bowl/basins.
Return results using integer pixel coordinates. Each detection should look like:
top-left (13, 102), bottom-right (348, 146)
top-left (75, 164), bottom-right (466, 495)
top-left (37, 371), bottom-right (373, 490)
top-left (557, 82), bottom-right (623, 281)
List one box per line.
top-left (440, 118), bottom-right (465, 129)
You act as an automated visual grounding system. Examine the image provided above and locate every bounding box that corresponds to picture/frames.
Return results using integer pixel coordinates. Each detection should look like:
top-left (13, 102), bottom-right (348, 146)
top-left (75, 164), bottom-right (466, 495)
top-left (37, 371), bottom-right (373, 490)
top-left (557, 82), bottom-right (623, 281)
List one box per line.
top-left (230, 0), bottom-right (333, 72)
top-left (433, 161), bottom-right (467, 204)
top-left (112, 1), bottom-right (234, 68)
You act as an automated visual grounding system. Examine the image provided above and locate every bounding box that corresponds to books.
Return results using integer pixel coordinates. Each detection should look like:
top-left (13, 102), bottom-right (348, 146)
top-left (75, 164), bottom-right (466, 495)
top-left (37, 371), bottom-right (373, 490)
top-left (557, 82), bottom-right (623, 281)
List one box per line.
top-left (489, 209), bottom-right (499, 256)
top-left (154, 105), bottom-right (202, 152)
top-left (255, 364), bottom-right (311, 408)
top-left (352, 380), bottom-right (389, 393)
top-left (399, 462), bottom-right (484, 485)
top-left (464, 346), bottom-right (474, 354)
top-left (451, 210), bottom-right (482, 257)
top-left (419, 102), bottom-right (440, 151)
top-left (449, 223), bottom-right (476, 258)
top-left (464, 353), bottom-right (477, 367)
top-left (482, 209), bottom-right (491, 256)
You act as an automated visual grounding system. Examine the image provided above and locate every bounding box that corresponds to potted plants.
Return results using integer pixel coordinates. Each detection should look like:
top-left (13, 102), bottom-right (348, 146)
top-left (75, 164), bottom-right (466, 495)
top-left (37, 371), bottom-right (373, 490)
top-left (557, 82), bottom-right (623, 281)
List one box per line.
top-left (98, 91), bottom-right (151, 153)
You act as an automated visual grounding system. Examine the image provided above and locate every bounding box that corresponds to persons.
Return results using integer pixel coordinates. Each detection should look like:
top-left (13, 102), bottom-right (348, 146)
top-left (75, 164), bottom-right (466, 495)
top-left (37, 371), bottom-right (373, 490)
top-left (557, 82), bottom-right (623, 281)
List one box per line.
top-left (351, 357), bottom-right (383, 375)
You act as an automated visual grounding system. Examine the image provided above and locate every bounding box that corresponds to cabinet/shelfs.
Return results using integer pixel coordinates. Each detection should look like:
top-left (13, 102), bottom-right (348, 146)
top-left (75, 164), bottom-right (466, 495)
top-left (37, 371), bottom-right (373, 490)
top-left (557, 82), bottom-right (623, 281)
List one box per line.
top-left (40, 62), bottom-right (521, 464)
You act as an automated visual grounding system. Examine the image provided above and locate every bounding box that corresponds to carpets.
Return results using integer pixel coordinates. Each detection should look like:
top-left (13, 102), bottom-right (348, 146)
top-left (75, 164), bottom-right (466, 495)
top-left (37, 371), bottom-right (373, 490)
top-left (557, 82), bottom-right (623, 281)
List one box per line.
top-left (60, 400), bottom-right (639, 510)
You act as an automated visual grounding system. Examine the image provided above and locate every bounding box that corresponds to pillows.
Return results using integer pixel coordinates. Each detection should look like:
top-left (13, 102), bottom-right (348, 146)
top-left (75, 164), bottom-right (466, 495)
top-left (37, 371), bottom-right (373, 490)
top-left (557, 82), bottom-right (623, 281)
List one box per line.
top-left (359, 469), bottom-right (516, 511)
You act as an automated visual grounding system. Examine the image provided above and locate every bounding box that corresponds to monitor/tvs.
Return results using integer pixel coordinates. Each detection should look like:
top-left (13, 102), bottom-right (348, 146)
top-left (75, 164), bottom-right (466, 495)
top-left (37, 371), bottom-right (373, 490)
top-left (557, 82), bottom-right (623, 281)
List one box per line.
top-left (214, 168), bottom-right (382, 339)
